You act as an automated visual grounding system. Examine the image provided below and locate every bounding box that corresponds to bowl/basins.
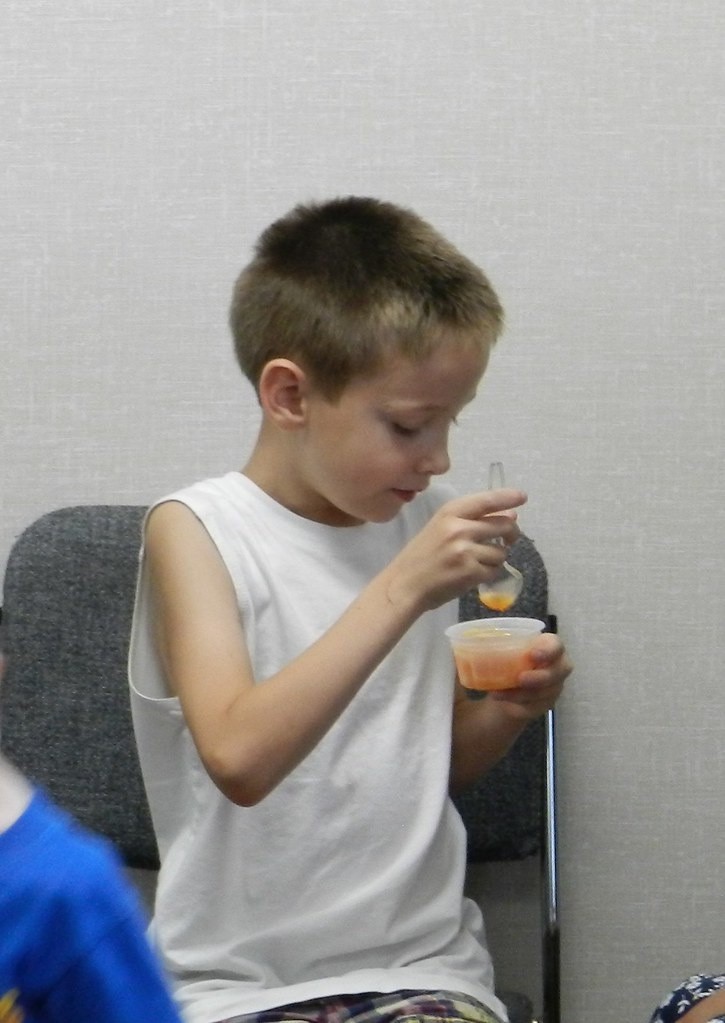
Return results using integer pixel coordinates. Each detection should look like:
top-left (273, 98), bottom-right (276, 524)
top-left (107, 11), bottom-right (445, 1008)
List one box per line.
top-left (445, 617), bottom-right (547, 692)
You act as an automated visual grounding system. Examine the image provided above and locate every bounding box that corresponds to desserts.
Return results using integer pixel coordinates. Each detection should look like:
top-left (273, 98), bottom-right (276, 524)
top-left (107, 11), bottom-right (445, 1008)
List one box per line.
top-left (444, 616), bottom-right (547, 690)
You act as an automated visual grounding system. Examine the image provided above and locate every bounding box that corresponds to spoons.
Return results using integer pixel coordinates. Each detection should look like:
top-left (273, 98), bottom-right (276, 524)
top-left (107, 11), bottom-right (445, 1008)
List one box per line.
top-left (477, 464), bottom-right (526, 611)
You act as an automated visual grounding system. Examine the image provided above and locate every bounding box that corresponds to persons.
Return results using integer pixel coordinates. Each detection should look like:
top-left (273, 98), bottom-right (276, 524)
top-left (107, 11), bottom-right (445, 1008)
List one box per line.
top-left (0, 752), bottom-right (183, 1023)
top-left (125, 199), bottom-right (576, 1023)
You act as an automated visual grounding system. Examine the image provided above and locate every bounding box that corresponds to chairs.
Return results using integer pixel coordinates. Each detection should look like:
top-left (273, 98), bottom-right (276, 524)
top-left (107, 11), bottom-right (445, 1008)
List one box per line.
top-left (0, 505), bottom-right (561, 1023)
